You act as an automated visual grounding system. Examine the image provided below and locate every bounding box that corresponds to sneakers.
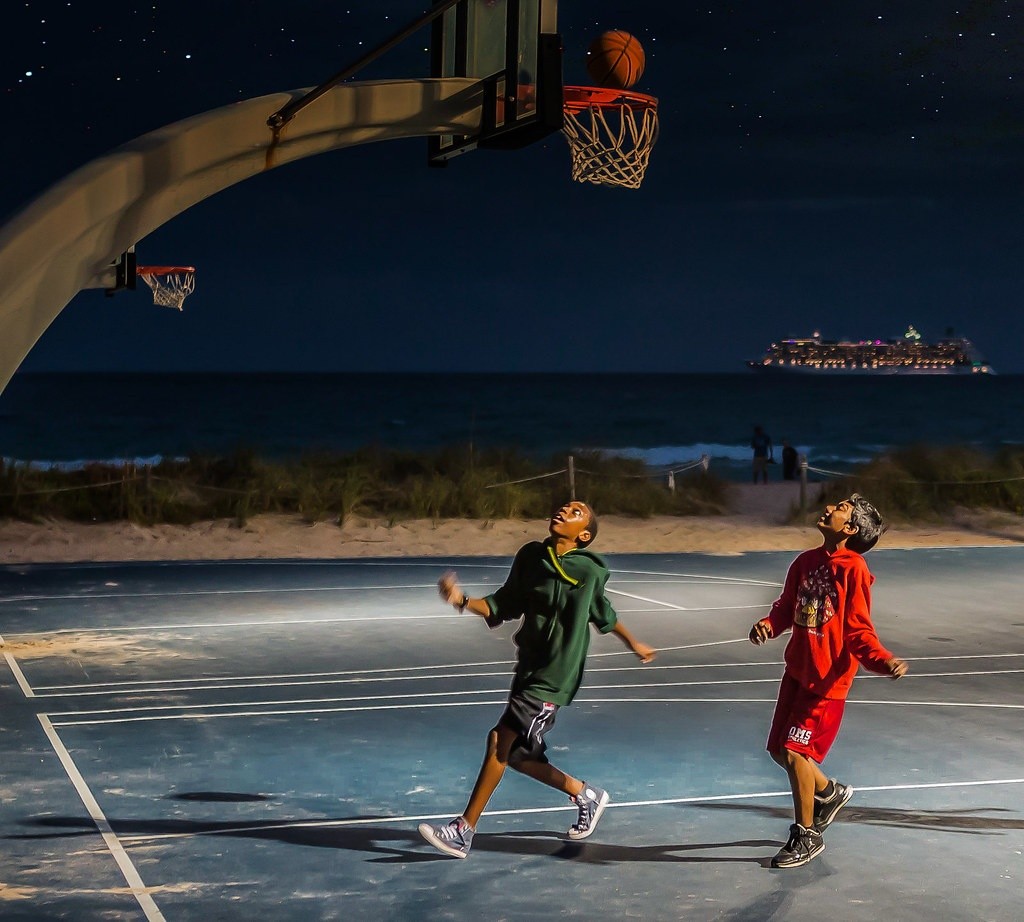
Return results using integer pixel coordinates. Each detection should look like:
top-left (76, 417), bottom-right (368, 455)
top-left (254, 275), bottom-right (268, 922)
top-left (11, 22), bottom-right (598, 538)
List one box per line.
top-left (813, 778), bottom-right (854, 834)
top-left (771, 823), bottom-right (825, 868)
top-left (567, 781), bottom-right (609, 839)
top-left (417, 816), bottom-right (476, 858)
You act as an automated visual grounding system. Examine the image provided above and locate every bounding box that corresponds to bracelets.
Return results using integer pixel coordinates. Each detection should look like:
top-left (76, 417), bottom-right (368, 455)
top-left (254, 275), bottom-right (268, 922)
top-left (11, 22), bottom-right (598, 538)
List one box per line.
top-left (458, 596), bottom-right (469, 608)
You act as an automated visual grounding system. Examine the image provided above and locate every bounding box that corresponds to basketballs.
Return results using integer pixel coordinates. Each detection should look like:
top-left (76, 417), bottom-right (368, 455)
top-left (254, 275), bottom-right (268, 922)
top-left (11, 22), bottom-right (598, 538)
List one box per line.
top-left (587, 29), bottom-right (646, 89)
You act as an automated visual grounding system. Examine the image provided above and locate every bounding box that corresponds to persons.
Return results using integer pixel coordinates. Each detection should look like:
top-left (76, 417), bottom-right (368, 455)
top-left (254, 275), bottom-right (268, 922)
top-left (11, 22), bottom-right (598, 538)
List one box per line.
top-left (782, 440), bottom-right (800, 480)
top-left (751, 426), bottom-right (773, 484)
top-left (749, 493), bottom-right (909, 868)
top-left (419, 502), bottom-right (656, 861)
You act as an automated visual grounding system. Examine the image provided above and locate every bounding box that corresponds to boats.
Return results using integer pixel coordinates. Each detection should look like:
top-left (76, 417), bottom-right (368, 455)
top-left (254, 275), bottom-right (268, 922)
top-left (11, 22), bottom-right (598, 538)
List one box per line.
top-left (743, 307), bottom-right (997, 394)
